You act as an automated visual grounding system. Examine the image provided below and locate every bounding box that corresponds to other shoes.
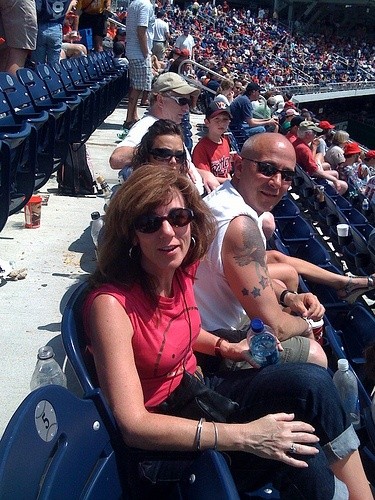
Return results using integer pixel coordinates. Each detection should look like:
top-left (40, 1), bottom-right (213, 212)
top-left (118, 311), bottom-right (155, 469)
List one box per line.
top-left (123, 121), bottom-right (135, 130)
top-left (190, 106), bottom-right (203, 114)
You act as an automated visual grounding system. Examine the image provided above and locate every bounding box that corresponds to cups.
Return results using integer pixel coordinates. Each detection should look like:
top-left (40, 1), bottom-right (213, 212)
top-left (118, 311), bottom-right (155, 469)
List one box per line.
top-left (71, 30), bottom-right (77, 37)
top-left (314, 185), bottom-right (325, 202)
top-left (337, 223), bottom-right (349, 237)
top-left (23, 196), bottom-right (42, 229)
top-left (306, 318), bottom-right (325, 348)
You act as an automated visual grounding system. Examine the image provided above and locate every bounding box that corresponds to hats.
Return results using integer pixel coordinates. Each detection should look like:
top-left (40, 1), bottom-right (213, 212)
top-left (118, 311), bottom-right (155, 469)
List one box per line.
top-left (183, 24), bottom-right (190, 32)
top-left (319, 121), bottom-right (335, 129)
top-left (153, 72), bottom-right (201, 97)
top-left (290, 117), bottom-right (305, 127)
top-left (206, 101), bottom-right (233, 120)
top-left (344, 143), bottom-right (361, 155)
top-left (285, 101), bottom-right (297, 109)
top-left (246, 81), bottom-right (266, 91)
top-left (366, 150), bottom-right (375, 158)
top-left (300, 121), bottom-right (323, 133)
top-left (66, 11), bottom-right (77, 18)
top-left (179, 49), bottom-right (191, 56)
top-left (267, 97), bottom-right (277, 104)
top-left (308, 111), bottom-right (316, 117)
top-left (285, 109), bottom-right (299, 116)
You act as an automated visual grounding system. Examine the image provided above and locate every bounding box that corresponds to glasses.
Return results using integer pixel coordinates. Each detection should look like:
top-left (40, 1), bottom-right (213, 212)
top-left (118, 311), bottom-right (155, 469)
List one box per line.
top-left (242, 157), bottom-right (295, 181)
top-left (236, 87), bottom-right (242, 91)
top-left (165, 92), bottom-right (191, 106)
top-left (128, 208), bottom-right (196, 234)
top-left (148, 147), bottom-right (184, 163)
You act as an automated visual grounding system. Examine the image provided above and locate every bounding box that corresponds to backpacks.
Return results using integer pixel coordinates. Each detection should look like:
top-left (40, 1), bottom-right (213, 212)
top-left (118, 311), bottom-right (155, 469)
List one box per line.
top-left (57, 140), bottom-right (103, 196)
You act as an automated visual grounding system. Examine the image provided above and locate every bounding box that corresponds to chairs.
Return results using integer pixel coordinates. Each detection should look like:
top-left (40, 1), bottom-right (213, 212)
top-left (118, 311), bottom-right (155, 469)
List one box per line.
top-left (0, 49), bottom-right (375, 500)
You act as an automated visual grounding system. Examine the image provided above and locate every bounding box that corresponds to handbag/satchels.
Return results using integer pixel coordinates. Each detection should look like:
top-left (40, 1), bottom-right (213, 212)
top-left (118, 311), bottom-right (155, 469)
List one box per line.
top-left (155, 371), bottom-right (240, 466)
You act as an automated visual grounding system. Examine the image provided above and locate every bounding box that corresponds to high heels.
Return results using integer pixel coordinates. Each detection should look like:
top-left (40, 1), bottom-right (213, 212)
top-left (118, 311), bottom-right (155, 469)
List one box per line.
top-left (336, 273), bottom-right (375, 304)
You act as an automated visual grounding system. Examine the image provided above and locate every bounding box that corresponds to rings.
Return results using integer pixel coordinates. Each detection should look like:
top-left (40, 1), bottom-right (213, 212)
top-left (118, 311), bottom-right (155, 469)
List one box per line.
top-left (291, 443), bottom-right (296, 455)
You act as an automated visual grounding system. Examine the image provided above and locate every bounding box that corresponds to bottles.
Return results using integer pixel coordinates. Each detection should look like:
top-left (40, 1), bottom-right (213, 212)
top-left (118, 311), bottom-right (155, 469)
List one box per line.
top-left (91, 211), bottom-right (105, 251)
top-left (362, 199), bottom-right (369, 210)
top-left (29, 346), bottom-right (67, 392)
top-left (246, 317), bottom-right (280, 367)
top-left (333, 358), bottom-right (358, 427)
top-left (96, 175), bottom-right (114, 207)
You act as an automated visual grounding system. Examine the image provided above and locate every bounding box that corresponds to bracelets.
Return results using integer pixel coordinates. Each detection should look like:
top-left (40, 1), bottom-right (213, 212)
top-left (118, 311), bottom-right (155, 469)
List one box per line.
top-left (212, 421), bottom-right (219, 450)
top-left (280, 290), bottom-right (297, 307)
top-left (196, 417), bottom-right (205, 453)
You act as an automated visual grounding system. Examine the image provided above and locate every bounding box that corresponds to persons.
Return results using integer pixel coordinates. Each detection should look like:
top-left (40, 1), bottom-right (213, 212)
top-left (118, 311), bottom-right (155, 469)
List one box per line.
top-left (0, 0), bottom-right (375, 500)
